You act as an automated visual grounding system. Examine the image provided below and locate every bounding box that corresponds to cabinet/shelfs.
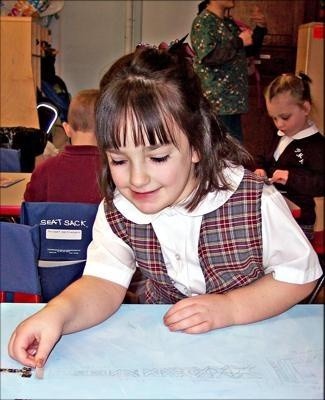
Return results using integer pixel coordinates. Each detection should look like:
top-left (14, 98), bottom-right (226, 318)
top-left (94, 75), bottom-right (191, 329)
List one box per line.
top-left (0, 15), bottom-right (52, 129)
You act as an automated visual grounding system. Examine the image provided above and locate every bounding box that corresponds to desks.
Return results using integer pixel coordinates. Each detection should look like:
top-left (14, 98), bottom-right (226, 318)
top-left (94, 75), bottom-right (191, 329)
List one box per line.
top-left (0, 303), bottom-right (324, 400)
top-left (0, 172), bottom-right (301, 217)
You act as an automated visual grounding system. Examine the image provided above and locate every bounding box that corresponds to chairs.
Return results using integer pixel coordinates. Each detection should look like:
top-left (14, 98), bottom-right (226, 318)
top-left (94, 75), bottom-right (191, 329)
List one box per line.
top-left (309, 230), bottom-right (325, 304)
top-left (0, 222), bottom-right (41, 303)
top-left (21, 202), bottom-right (98, 303)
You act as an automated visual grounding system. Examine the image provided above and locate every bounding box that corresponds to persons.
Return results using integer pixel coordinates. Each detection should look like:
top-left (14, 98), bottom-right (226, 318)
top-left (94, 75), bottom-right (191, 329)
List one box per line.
top-left (8, 45), bottom-right (323, 369)
top-left (191, 0), bottom-right (267, 147)
top-left (254, 70), bottom-right (325, 274)
top-left (24, 90), bottom-right (109, 204)
